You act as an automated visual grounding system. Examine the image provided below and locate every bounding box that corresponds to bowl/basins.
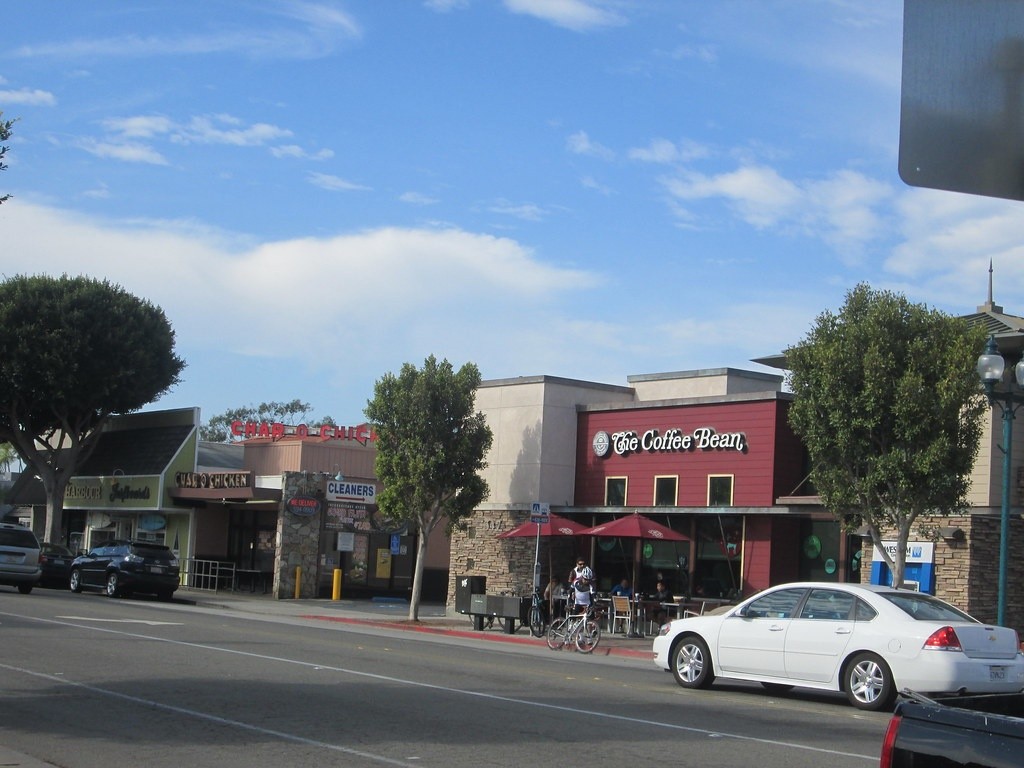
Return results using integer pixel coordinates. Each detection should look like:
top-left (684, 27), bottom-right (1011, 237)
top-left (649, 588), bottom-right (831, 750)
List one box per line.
top-left (597, 592), bottom-right (608, 598)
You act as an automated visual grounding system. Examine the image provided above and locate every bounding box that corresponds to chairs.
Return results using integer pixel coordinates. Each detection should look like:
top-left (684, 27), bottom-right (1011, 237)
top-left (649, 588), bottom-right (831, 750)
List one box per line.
top-left (612, 595), bottom-right (721, 637)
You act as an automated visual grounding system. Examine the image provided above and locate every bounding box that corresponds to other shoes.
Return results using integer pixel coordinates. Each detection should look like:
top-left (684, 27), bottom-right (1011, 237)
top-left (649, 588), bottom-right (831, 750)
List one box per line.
top-left (586, 636), bottom-right (592, 644)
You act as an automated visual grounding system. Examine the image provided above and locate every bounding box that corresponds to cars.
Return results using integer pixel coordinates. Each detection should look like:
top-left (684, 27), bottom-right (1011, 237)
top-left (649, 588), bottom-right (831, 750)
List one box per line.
top-left (653, 580), bottom-right (1024, 712)
top-left (39, 542), bottom-right (75, 589)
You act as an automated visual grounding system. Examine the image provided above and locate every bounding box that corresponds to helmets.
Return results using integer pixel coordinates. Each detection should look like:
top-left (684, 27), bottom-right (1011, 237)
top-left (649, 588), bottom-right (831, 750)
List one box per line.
top-left (581, 566), bottom-right (593, 580)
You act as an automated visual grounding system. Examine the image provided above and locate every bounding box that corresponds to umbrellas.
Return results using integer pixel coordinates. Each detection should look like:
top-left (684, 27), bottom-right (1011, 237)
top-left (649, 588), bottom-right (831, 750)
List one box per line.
top-left (575, 509), bottom-right (691, 601)
top-left (495, 513), bottom-right (589, 613)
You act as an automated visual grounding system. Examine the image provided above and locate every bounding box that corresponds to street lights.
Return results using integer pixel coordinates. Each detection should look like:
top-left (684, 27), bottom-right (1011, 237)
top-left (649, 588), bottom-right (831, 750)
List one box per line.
top-left (973, 335), bottom-right (1024, 630)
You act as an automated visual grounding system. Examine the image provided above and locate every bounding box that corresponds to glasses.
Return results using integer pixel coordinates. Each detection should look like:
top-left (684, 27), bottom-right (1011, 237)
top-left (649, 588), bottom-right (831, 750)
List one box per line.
top-left (578, 563), bottom-right (586, 565)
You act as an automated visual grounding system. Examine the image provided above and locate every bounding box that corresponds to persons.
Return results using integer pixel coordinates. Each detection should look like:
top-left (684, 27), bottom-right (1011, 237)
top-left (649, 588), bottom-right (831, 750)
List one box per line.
top-left (570, 556), bottom-right (593, 620)
top-left (544, 574), bottom-right (564, 600)
top-left (693, 585), bottom-right (705, 598)
top-left (651, 582), bottom-right (673, 626)
top-left (611, 579), bottom-right (633, 632)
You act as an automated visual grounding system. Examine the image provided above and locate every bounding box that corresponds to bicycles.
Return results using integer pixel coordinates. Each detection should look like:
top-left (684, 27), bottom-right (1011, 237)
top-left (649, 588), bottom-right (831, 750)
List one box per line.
top-left (527, 592), bottom-right (547, 638)
top-left (546, 593), bottom-right (601, 654)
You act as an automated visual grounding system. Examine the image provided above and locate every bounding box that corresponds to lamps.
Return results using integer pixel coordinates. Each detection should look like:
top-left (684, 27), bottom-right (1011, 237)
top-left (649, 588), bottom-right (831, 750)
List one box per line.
top-left (110, 469), bottom-right (128, 486)
top-left (330, 464), bottom-right (345, 481)
top-left (933, 528), bottom-right (962, 540)
top-left (850, 525), bottom-right (880, 537)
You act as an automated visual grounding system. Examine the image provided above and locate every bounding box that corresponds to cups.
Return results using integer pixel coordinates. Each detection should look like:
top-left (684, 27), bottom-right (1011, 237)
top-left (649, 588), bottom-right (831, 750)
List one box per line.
top-left (635, 593), bottom-right (639, 602)
top-left (560, 588), bottom-right (571, 597)
top-left (606, 592), bottom-right (621, 598)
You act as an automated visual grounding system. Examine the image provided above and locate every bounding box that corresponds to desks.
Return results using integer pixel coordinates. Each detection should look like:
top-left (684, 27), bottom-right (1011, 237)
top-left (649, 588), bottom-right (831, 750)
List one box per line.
top-left (551, 595), bottom-right (573, 627)
top-left (600, 598), bottom-right (701, 636)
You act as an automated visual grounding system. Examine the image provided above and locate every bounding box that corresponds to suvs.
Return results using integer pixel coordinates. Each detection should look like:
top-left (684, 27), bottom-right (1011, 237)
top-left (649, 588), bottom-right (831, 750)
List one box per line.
top-left (0, 522), bottom-right (43, 594)
top-left (68, 538), bottom-right (180, 603)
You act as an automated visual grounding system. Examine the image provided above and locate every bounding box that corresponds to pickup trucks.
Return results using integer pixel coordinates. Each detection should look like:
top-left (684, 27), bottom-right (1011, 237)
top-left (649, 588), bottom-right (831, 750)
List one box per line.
top-left (881, 689), bottom-right (1023, 768)
